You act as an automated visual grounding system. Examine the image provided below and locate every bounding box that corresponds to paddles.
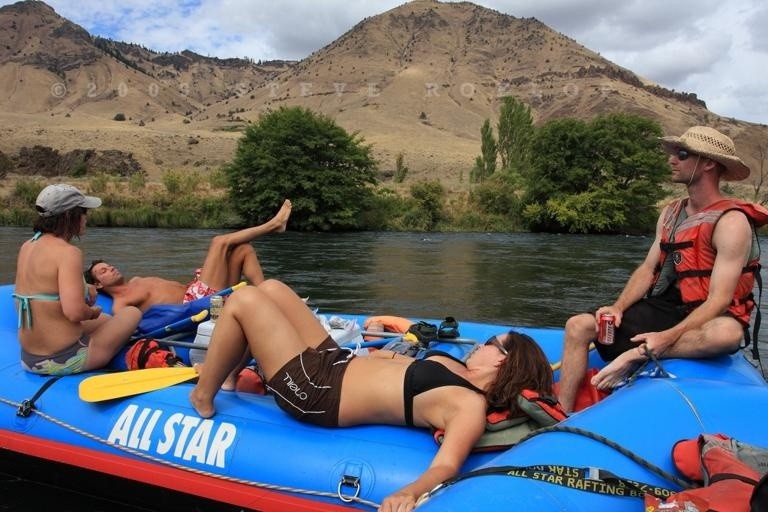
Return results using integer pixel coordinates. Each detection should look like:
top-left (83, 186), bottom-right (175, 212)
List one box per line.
top-left (79, 332), bottom-right (418, 403)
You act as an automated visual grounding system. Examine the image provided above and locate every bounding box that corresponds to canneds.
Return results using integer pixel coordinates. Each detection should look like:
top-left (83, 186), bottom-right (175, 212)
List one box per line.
top-left (596, 315), bottom-right (617, 346)
top-left (209, 294), bottom-right (223, 323)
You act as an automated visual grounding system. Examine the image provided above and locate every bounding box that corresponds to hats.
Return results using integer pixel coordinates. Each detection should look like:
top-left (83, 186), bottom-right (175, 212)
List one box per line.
top-left (656, 125), bottom-right (751, 182)
top-left (35, 184), bottom-right (103, 218)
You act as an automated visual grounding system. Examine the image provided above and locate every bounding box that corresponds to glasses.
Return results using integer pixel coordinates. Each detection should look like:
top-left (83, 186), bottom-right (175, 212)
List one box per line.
top-left (676, 150), bottom-right (689, 161)
top-left (484, 336), bottom-right (509, 356)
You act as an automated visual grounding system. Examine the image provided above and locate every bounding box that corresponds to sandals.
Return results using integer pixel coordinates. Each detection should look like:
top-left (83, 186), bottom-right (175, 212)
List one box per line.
top-left (408, 320), bottom-right (437, 344)
top-left (438, 317), bottom-right (461, 339)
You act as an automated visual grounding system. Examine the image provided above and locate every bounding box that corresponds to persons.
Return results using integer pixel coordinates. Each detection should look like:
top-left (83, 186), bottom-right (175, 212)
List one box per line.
top-left (189, 277), bottom-right (555, 512)
top-left (553, 124), bottom-right (766, 416)
top-left (82, 198), bottom-right (293, 321)
top-left (12, 184), bottom-right (144, 376)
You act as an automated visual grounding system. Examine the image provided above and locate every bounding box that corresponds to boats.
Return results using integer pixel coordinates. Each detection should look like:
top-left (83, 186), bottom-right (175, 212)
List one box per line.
top-left (0, 282), bottom-right (768, 512)
